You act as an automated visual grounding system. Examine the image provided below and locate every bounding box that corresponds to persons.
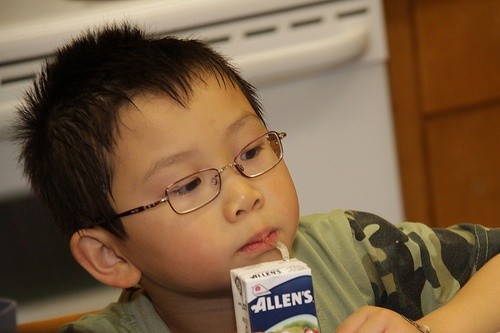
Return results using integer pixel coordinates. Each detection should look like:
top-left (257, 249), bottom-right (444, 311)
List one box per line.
top-left (11, 20), bottom-right (500, 333)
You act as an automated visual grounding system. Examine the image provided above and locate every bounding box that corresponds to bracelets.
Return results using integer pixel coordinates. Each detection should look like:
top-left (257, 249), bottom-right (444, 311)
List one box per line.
top-left (402, 315), bottom-right (430, 333)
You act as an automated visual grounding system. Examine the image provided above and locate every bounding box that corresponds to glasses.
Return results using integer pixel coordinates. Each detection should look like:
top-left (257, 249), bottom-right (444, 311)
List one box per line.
top-left (87, 130), bottom-right (288, 230)
